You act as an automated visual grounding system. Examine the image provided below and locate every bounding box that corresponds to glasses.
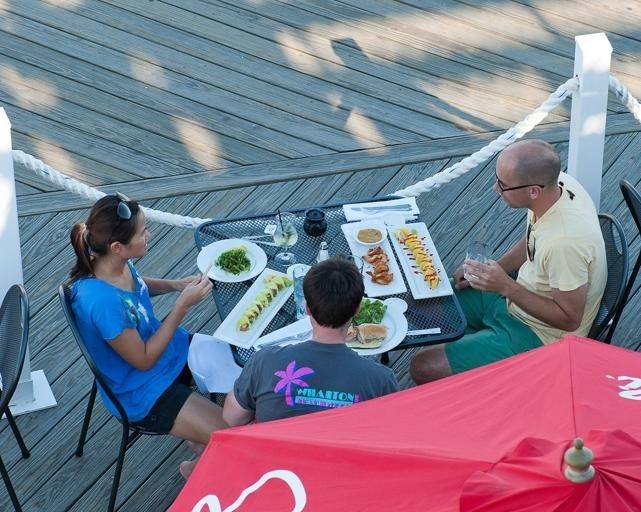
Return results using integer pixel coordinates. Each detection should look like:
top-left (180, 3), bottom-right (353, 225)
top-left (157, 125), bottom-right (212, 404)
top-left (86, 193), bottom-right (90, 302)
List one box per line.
top-left (495, 169), bottom-right (544, 192)
top-left (106, 192), bottom-right (132, 244)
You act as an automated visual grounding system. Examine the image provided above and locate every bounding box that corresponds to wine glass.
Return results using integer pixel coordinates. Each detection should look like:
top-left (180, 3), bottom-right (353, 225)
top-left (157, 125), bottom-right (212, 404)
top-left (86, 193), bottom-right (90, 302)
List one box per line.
top-left (273, 213), bottom-right (298, 269)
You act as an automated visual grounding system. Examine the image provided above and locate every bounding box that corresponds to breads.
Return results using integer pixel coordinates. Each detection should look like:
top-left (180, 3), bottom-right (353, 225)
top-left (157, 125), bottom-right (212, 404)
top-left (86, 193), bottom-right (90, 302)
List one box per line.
top-left (356, 324), bottom-right (386, 343)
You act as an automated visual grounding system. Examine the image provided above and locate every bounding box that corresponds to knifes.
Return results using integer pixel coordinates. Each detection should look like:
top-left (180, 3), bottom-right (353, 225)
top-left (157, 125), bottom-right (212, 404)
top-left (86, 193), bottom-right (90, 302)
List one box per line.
top-left (348, 203), bottom-right (412, 213)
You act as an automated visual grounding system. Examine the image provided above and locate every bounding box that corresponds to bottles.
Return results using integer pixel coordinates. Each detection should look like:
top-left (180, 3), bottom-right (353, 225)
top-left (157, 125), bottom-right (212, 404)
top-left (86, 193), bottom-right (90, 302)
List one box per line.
top-left (317, 241), bottom-right (330, 264)
top-left (303, 207), bottom-right (327, 237)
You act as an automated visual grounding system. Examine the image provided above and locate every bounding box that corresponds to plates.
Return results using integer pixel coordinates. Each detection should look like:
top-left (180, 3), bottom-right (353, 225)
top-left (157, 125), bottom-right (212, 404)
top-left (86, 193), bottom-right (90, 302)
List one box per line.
top-left (344, 297), bottom-right (408, 356)
top-left (341, 221), bottom-right (454, 300)
top-left (196, 238), bottom-right (294, 352)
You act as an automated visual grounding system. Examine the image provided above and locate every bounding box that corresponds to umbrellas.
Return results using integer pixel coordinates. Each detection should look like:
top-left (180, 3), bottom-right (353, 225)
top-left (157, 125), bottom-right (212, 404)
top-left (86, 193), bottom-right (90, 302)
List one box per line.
top-left (165, 335), bottom-right (641, 512)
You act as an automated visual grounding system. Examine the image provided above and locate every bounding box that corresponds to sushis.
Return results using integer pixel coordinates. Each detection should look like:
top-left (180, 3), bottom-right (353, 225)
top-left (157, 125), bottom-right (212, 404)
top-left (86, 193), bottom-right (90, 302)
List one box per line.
top-left (404, 232), bottom-right (439, 287)
top-left (237, 277), bottom-right (284, 331)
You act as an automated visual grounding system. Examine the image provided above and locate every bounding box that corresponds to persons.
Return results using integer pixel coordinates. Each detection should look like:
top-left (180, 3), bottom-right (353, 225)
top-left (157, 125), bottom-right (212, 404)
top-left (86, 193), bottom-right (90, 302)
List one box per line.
top-left (222, 257), bottom-right (400, 427)
top-left (67, 195), bottom-right (230, 483)
top-left (409, 138), bottom-right (608, 385)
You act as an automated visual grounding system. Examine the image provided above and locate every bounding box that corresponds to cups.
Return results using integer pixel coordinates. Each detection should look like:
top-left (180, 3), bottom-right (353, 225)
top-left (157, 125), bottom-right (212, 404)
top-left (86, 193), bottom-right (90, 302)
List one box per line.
top-left (463, 242), bottom-right (489, 282)
top-left (293, 265), bottom-right (313, 319)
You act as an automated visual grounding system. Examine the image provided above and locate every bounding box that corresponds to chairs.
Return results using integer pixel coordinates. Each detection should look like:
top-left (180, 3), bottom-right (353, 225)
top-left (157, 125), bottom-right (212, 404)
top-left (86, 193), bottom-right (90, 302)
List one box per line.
top-left (587, 212), bottom-right (629, 344)
top-left (0, 282), bottom-right (30, 511)
top-left (604, 177), bottom-right (640, 344)
top-left (55, 282), bottom-right (218, 512)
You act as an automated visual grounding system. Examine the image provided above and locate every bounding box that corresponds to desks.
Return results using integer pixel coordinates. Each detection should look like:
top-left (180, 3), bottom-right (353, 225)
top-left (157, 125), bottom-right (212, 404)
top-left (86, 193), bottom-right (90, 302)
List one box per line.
top-left (194, 195), bottom-right (467, 367)
